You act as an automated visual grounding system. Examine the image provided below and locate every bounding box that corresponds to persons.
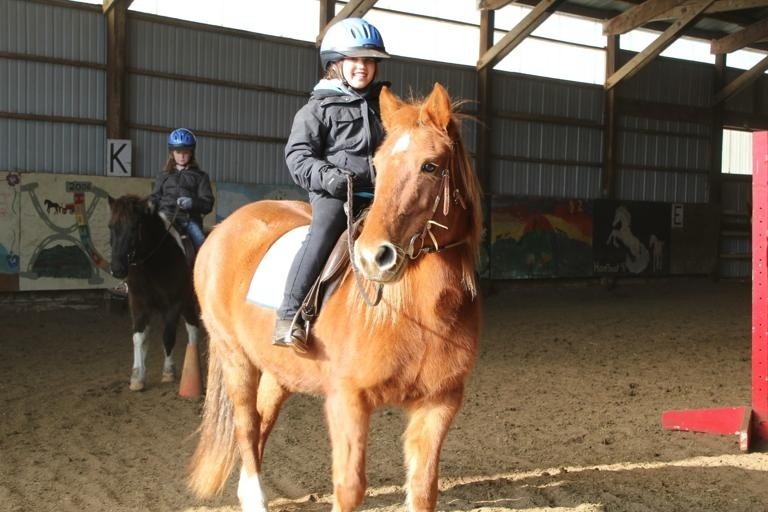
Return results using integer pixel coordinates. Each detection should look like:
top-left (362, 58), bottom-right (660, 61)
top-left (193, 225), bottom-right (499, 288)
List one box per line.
top-left (152, 127), bottom-right (215, 252)
top-left (273, 17), bottom-right (391, 350)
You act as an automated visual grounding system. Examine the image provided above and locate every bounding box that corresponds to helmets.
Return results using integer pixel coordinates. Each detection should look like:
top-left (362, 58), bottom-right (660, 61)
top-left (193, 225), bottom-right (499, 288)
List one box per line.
top-left (168, 129), bottom-right (195, 150)
top-left (321, 18), bottom-right (391, 69)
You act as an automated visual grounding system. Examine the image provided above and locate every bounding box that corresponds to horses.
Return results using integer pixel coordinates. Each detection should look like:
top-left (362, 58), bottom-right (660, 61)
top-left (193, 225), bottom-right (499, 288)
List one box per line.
top-left (105, 192), bottom-right (210, 392)
top-left (185, 83), bottom-right (484, 512)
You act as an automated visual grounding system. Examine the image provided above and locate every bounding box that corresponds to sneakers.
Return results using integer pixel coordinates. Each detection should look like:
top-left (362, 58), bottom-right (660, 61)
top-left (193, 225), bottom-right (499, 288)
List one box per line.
top-left (273, 320), bottom-right (306, 347)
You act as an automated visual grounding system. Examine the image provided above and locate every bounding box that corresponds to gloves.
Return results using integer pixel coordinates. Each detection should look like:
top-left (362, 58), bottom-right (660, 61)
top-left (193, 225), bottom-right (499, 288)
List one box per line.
top-left (321, 165), bottom-right (354, 195)
top-left (177, 197), bottom-right (191, 208)
